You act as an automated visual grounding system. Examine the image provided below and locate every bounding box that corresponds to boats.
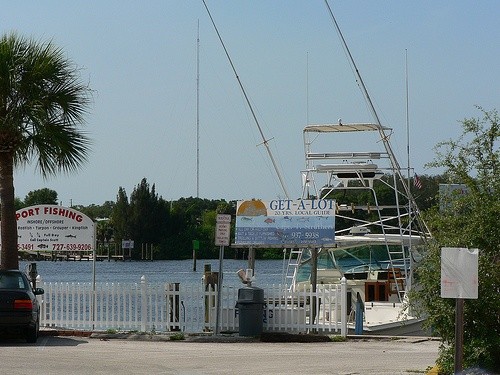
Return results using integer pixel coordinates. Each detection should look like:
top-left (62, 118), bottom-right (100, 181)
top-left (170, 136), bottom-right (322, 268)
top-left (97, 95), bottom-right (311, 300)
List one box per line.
top-left (280, 118), bottom-right (438, 337)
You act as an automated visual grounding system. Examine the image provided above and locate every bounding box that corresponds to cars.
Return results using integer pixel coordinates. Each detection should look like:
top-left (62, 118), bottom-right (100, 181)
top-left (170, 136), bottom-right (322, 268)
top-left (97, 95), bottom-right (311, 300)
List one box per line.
top-left (0, 268), bottom-right (45, 343)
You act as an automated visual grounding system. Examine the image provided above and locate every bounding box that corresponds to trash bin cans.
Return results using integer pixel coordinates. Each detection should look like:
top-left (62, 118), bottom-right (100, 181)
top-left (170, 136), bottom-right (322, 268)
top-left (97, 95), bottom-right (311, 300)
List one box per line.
top-left (234, 286), bottom-right (265, 337)
top-left (365, 281), bottom-right (389, 304)
